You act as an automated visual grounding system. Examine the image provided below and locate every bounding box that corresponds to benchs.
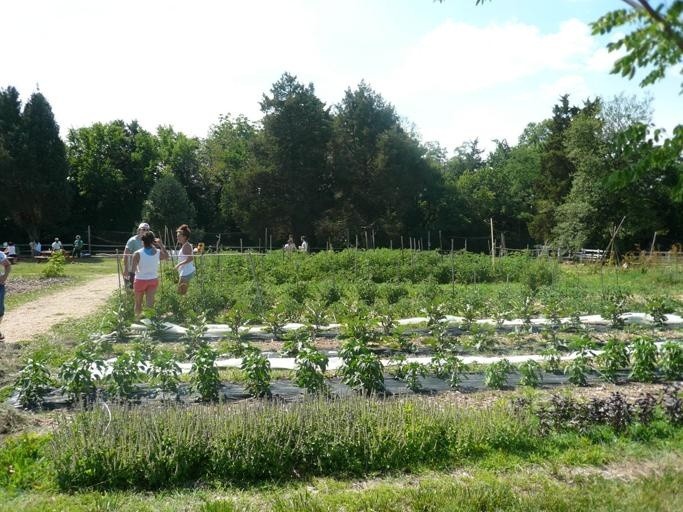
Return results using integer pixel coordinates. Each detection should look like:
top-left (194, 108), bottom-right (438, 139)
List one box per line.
top-left (35, 256), bottom-right (73, 263)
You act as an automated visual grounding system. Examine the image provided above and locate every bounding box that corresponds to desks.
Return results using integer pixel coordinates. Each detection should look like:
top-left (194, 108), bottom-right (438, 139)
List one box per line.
top-left (41, 251), bottom-right (71, 263)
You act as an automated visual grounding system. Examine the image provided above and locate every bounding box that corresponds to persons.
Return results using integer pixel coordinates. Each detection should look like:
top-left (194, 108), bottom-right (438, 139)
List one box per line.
top-left (172, 225), bottom-right (195, 294)
top-left (32, 238), bottom-right (41, 256)
top-left (285, 239), bottom-right (294, 248)
top-left (72, 235), bottom-right (84, 258)
top-left (131, 230), bottom-right (169, 315)
top-left (123, 222), bottom-right (150, 294)
top-left (0, 250), bottom-right (11, 341)
top-left (51, 238), bottom-right (64, 251)
top-left (4, 241), bottom-right (16, 257)
top-left (298, 236), bottom-right (309, 251)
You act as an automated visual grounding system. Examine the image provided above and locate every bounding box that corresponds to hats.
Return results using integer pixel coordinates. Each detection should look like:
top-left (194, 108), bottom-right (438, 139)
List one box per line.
top-left (138, 222), bottom-right (150, 229)
top-left (76, 235), bottom-right (80, 239)
top-left (55, 238), bottom-right (59, 240)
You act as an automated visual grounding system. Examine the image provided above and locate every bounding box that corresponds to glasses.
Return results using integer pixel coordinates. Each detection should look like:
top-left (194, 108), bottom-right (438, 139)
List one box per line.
top-left (143, 229), bottom-right (148, 231)
